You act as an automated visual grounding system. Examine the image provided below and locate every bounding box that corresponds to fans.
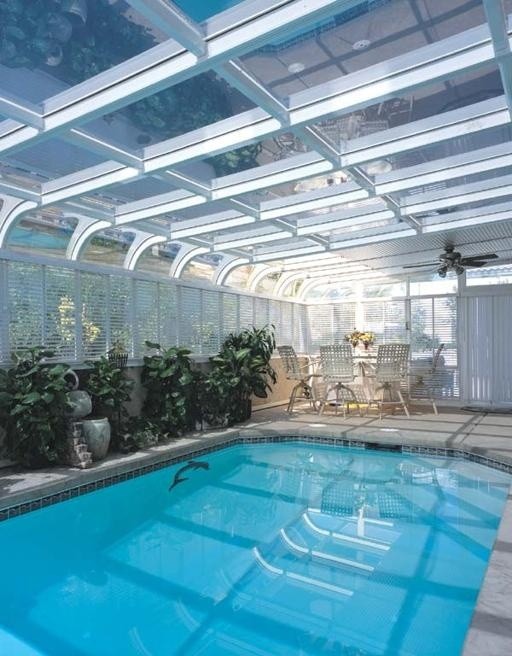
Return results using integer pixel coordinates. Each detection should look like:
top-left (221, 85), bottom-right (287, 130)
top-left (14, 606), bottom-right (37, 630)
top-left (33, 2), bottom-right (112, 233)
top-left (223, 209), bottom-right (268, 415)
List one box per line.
top-left (400, 241), bottom-right (503, 280)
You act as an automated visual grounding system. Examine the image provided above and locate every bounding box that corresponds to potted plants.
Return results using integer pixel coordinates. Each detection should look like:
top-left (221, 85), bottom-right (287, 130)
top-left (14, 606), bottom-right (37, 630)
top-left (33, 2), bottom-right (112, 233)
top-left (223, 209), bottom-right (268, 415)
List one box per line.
top-left (2, 319), bottom-right (276, 470)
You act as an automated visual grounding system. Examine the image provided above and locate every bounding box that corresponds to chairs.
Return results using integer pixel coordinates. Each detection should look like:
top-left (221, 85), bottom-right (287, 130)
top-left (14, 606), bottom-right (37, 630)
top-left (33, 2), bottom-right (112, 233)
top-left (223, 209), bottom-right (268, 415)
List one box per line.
top-left (277, 338), bottom-right (445, 426)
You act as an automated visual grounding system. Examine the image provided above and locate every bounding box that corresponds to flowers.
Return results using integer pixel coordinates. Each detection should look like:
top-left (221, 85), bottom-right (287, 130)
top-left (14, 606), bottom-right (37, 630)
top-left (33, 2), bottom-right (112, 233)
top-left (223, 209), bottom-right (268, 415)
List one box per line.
top-left (342, 330), bottom-right (375, 342)
